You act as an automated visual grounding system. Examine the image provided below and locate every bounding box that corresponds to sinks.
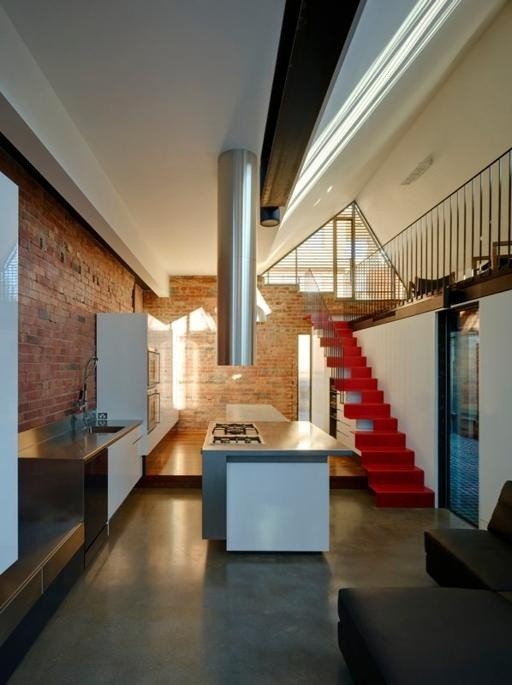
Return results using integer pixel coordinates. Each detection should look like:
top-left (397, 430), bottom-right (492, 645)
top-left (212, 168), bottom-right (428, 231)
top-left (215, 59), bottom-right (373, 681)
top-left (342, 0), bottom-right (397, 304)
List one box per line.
top-left (81, 426), bottom-right (125, 434)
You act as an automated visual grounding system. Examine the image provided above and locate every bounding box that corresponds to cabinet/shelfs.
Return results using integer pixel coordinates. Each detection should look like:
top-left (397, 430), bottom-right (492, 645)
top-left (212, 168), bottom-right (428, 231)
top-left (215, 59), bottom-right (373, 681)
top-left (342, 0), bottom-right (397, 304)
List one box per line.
top-left (108, 424), bottom-right (145, 536)
top-left (19, 448), bottom-right (109, 567)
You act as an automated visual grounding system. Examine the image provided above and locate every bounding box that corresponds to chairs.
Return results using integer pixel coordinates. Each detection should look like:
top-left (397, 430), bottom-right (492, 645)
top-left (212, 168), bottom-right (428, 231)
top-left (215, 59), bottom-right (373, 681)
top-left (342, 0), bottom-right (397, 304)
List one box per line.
top-left (332, 480), bottom-right (510, 685)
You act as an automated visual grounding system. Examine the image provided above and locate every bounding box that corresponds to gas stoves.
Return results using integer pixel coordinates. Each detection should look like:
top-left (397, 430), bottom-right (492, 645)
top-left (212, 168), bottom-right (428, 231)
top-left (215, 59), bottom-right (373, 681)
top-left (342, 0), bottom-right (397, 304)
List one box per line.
top-left (208, 419), bottom-right (266, 448)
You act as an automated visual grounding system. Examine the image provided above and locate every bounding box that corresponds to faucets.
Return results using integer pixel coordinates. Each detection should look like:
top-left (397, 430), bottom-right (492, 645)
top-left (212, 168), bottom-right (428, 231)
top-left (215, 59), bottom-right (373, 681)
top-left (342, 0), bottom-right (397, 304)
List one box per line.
top-left (79, 356), bottom-right (98, 401)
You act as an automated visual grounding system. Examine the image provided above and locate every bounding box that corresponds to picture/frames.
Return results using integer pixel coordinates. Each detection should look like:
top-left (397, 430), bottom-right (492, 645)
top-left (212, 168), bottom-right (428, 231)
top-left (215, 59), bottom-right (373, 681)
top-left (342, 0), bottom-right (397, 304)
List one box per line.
top-left (147, 350), bottom-right (160, 431)
